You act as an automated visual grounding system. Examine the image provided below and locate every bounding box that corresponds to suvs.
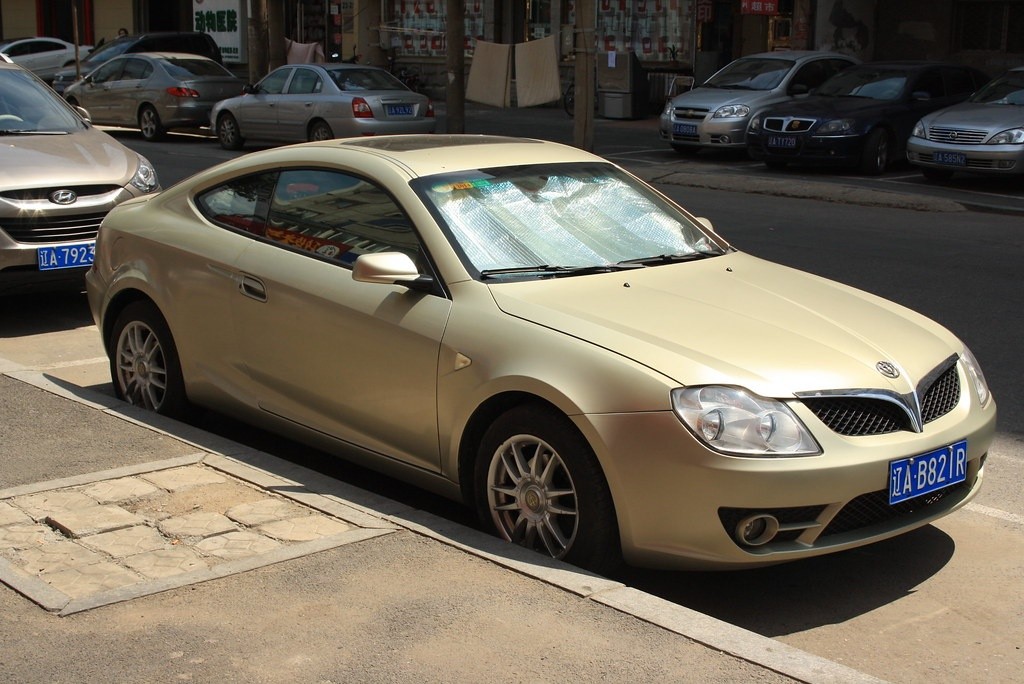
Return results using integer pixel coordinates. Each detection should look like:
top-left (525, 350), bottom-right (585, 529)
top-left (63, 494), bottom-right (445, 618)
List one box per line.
top-left (53, 30), bottom-right (224, 97)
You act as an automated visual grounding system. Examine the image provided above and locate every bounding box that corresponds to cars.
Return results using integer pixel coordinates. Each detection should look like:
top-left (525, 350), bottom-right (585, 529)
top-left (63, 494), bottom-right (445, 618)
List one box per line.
top-left (210, 63), bottom-right (438, 151)
top-left (0, 52), bottom-right (163, 271)
top-left (907, 67), bottom-right (1024, 189)
top-left (63, 52), bottom-right (245, 141)
top-left (0, 37), bottom-right (95, 88)
top-left (747, 61), bottom-right (993, 175)
top-left (85, 134), bottom-right (997, 579)
top-left (659, 50), bottom-right (862, 159)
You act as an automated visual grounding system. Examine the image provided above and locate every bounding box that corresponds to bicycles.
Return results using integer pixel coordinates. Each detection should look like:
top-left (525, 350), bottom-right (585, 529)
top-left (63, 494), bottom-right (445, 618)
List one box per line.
top-left (562, 67), bottom-right (598, 116)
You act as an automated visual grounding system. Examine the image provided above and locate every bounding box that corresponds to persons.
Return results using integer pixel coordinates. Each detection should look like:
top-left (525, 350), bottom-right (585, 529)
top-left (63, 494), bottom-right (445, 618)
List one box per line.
top-left (118, 28), bottom-right (128, 37)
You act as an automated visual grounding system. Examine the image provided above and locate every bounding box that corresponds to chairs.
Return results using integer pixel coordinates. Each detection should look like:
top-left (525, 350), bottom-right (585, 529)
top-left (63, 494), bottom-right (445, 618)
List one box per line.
top-left (664, 76), bottom-right (695, 107)
